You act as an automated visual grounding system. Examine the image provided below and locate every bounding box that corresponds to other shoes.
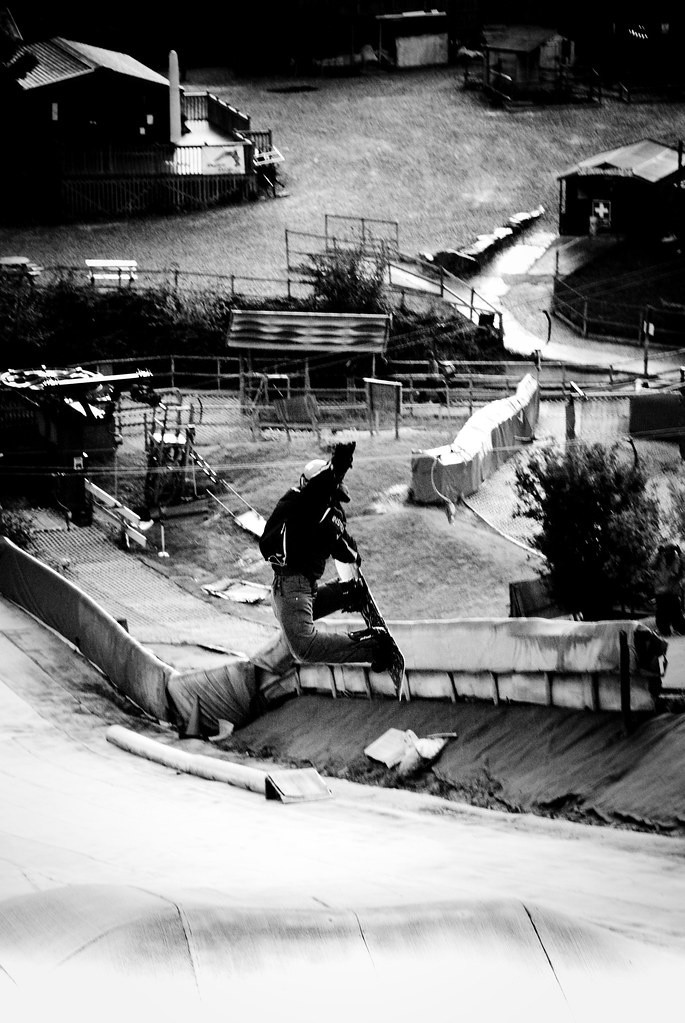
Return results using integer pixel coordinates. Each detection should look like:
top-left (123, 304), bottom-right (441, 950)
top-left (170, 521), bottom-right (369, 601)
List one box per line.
top-left (348, 625), bottom-right (390, 673)
top-left (327, 579), bottom-right (365, 613)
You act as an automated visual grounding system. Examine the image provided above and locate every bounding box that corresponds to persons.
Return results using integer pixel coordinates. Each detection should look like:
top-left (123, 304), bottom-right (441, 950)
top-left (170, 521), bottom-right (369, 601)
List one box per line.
top-left (260, 441), bottom-right (393, 675)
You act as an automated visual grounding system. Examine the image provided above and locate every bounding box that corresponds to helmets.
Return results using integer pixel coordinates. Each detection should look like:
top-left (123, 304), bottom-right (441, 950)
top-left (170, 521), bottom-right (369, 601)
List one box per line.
top-left (304, 459), bottom-right (330, 480)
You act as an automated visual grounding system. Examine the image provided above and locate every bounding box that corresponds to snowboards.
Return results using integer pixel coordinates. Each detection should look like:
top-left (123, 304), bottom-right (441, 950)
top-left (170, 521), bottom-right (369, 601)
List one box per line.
top-left (352, 567), bottom-right (407, 706)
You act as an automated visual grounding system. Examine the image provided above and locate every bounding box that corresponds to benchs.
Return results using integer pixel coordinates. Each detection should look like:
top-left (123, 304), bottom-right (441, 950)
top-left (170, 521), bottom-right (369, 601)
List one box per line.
top-left (0, 257), bottom-right (35, 287)
top-left (85, 266), bottom-right (139, 280)
top-left (1, 265), bottom-right (43, 277)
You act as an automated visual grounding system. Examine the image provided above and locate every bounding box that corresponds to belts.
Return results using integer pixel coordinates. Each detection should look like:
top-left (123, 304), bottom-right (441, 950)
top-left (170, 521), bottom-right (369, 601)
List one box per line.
top-left (275, 569), bottom-right (300, 576)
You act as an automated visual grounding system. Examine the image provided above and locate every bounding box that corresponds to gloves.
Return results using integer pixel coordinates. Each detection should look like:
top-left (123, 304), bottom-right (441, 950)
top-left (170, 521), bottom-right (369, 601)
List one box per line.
top-left (332, 441), bottom-right (358, 471)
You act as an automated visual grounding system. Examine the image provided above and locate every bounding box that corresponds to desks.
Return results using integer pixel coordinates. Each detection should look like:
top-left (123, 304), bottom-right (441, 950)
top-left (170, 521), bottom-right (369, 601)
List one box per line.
top-left (84, 259), bottom-right (137, 287)
